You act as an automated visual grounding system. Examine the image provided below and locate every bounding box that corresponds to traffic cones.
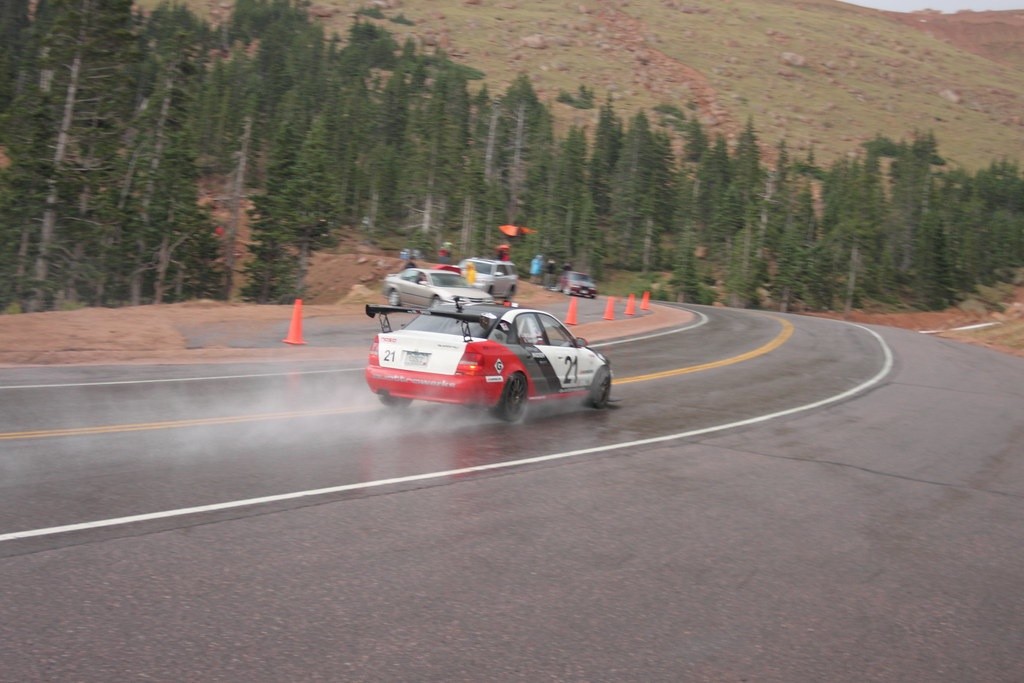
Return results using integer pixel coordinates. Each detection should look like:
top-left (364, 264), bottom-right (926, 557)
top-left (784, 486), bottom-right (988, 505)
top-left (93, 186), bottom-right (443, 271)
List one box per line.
top-left (624, 293), bottom-right (635, 315)
top-left (603, 296), bottom-right (615, 320)
top-left (281, 300), bottom-right (305, 345)
top-left (639, 291), bottom-right (650, 311)
top-left (564, 297), bottom-right (578, 325)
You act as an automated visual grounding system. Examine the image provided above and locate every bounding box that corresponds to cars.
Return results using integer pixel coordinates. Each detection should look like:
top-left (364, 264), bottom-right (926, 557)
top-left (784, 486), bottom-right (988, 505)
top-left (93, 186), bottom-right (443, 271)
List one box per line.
top-left (561, 271), bottom-right (598, 299)
top-left (455, 257), bottom-right (518, 298)
top-left (364, 296), bottom-right (612, 422)
top-left (381, 268), bottom-right (496, 309)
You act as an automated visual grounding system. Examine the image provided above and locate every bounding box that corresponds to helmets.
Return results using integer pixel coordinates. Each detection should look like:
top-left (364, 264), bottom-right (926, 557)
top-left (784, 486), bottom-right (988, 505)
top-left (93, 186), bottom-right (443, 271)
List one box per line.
top-left (442, 242), bottom-right (452, 246)
top-left (535, 255), bottom-right (543, 259)
top-left (547, 259), bottom-right (555, 264)
top-left (564, 263), bottom-right (572, 267)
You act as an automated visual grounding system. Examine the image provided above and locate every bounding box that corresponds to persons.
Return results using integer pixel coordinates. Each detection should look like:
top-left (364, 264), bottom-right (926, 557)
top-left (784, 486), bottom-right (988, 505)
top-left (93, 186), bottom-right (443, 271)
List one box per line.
top-left (499, 248), bottom-right (510, 275)
top-left (417, 272), bottom-right (427, 284)
top-left (405, 256), bottom-right (415, 268)
top-left (530, 255), bottom-right (543, 283)
top-left (466, 262), bottom-right (476, 284)
top-left (439, 243), bottom-right (452, 264)
top-left (554, 263), bottom-right (571, 292)
top-left (545, 260), bottom-right (557, 290)
